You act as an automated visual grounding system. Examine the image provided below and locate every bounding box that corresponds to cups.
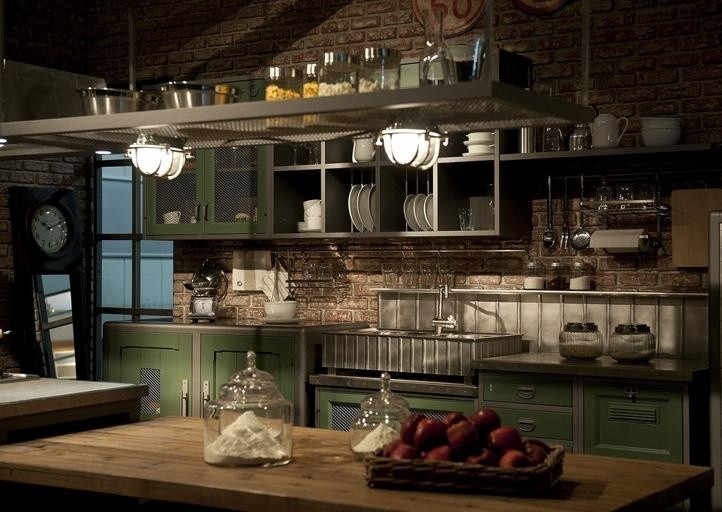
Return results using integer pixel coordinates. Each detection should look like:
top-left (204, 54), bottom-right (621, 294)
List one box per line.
top-left (465, 132), bottom-right (494, 140)
top-left (303, 200), bottom-right (322, 228)
top-left (380, 261), bottom-right (453, 290)
top-left (162, 210), bottom-right (182, 225)
top-left (468, 144), bottom-right (495, 153)
top-left (300, 262), bottom-right (347, 284)
top-left (351, 138), bottom-right (377, 163)
top-left (543, 126), bottom-right (564, 152)
top-left (519, 126), bottom-right (538, 154)
top-left (458, 207), bottom-right (474, 230)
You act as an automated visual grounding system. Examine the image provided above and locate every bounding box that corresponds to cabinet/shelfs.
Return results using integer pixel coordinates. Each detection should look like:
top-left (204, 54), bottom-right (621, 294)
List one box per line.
top-left (583, 378), bottom-right (710, 512)
top-left (141, 75), bottom-right (296, 240)
top-left (267, 128), bottom-right (500, 239)
top-left (479, 371), bottom-right (583, 454)
top-left (313, 386), bottom-right (477, 432)
top-left (104, 321), bottom-right (368, 427)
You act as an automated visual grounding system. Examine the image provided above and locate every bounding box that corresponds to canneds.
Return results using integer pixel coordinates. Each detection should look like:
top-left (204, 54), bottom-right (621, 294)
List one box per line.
top-left (264, 47), bottom-right (401, 128)
top-left (523, 261), bottom-right (591, 291)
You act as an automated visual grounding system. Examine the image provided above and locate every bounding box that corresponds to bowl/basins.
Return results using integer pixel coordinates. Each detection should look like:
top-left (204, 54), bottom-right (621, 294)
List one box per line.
top-left (637, 114), bottom-right (682, 145)
top-left (157, 81), bottom-right (242, 110)
top-left (262, 300), bottom-right (298, 319)
top-left (75, 86), bottom-right (158, 116)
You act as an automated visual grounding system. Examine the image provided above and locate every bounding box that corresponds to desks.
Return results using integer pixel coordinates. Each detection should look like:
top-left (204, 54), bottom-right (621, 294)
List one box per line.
top-left (0, 414), bottom-right (714, 512)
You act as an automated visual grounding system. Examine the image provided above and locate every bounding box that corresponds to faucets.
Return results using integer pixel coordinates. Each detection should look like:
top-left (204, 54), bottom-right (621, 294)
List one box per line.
top-left (431, 284), bottom-right (456, 335)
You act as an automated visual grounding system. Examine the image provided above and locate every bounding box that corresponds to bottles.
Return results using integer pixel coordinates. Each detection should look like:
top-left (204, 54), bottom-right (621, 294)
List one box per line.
top-left (418, 9), bottom-right (458, 88)
top-left (569, 123), bottom-right (591, 151)
top-left (524, 260), bottom-right (591, 290)
top-left (349, 372), bottom-right (410, 461)
top-left (263, 48), bottom-right (402, 128)
top-left (606, 323), bottom-right (656, 363)
top-left (594, 175), bottom-right (636, 211)
top-left (199, 350), bottom-right (298, 469)
top-left (559, 321), bottom-right (603, 360)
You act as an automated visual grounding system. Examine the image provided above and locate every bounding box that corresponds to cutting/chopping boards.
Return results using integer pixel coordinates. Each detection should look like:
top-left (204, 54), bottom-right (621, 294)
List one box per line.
top-left (671, 189), bottom-right (721, 267)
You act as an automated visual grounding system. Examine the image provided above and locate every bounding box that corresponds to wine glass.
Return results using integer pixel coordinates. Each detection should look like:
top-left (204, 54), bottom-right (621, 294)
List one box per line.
top-left (288, 140), bottom-right (320, 165)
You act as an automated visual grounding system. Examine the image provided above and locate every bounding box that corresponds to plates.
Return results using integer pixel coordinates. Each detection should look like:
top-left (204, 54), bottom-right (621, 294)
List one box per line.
top-left (412, 0), bottom-right (486, 39)
top-left (462, 152), bottom-right (494, 157)
top-left (463, 140), bottom-right (495, 146)
top-left (296, 222), bottom-right (320, 232)
top-left (260, 317), bottom-right (303, 324)
top-left (347, 182), bottom-right (378, 232)
top-left (402, 193), bottom-right (433, 230)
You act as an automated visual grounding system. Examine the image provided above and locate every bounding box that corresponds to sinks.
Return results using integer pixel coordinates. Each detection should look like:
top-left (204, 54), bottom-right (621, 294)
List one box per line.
top-left (335, 326), bottom-right (428, 336)
top-left (429, 331), bottom-right (510, 340)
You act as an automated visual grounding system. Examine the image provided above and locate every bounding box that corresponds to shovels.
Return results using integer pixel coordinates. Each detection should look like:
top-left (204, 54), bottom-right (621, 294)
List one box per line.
top-left (542, 175), bottom-right (556, 249)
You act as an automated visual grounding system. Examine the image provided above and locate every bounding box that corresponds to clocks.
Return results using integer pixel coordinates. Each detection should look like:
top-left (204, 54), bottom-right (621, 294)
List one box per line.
top-left (23, 197), bottom-right (76, 260)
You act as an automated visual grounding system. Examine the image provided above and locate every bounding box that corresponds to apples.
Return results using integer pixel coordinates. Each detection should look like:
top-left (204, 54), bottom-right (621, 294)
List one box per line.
top-left (381, 408), bottom-right (549, 468)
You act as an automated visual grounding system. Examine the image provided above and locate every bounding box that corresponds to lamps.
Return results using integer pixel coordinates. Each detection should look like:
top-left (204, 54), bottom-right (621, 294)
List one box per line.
top-left (378, 124), bottom-right (443, 170)
top-left (127, 131), bottom-right (186, 180)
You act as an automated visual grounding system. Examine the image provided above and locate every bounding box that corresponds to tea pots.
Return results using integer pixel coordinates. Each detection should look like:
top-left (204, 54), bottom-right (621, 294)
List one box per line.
top-left (589, 114), bottom-right (630, 149)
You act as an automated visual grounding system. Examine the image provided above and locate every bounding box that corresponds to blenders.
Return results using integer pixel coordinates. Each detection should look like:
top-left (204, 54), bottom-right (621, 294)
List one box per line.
top-left (184, 260), bottom-right (230, 322)
